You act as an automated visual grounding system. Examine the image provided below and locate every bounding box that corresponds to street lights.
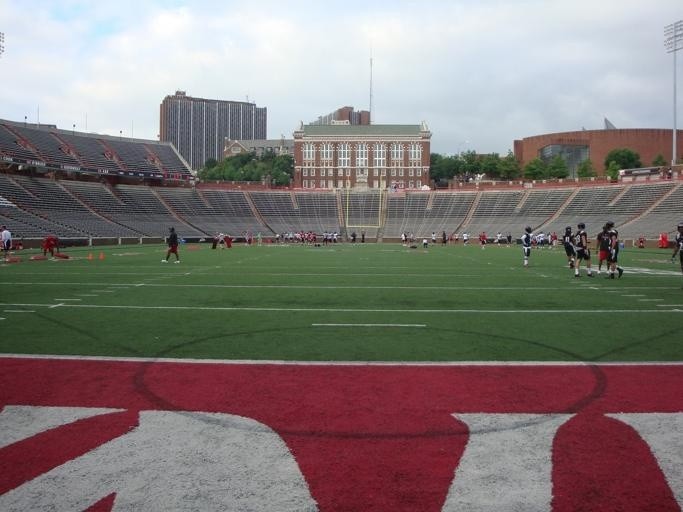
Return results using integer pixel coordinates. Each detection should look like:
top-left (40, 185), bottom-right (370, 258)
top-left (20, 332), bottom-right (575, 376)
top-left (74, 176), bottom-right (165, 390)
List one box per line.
top-left (664, 19), bottom-right (683, 164)
top-left (120, 131), bottom-right (122, 140)
top-left (0, 32), bottom-right (4, 55)
top-left (25, 116), bottom-right (27, 126)
top-left (73, 124), bottom-right (75, 136)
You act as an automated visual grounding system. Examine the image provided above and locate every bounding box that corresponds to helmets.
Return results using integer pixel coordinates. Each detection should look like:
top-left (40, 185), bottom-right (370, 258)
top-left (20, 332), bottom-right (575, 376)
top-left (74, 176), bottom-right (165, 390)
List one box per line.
top-left (603, 220), bottom-right (616, 231)
top-left (525, 226), bottom-right (531, 233)
top-left (566, 226), bottom-right (571, 232)
top-left (578, 223), bottom-right (585, 229)
top-left (678, 222), bottom-right (683, 233)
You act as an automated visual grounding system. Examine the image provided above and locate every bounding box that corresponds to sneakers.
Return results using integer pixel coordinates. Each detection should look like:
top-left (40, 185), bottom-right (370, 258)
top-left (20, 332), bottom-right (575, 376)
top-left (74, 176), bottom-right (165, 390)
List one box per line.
top-left (605, 267), bottom-right (623, 279)
top-left (568, 259), bottom-right (574, 268)
top-left (162, 260), bottom-right (180, 264)
top-left (574, 271), bottom-right (601, 277)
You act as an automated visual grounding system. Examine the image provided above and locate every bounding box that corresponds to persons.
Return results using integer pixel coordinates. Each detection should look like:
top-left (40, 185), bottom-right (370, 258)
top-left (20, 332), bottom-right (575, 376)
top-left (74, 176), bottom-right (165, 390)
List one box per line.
top-left (211, 230), bottom-right (357, 250)
top-left (0, 225), bottom-right (12, 259)
top-left (40, 236), bottom-right (66, 256)
top-left (161, 227), bottom-right (180, 264)
top-left (400, 221), bottom-right (683, 279)
